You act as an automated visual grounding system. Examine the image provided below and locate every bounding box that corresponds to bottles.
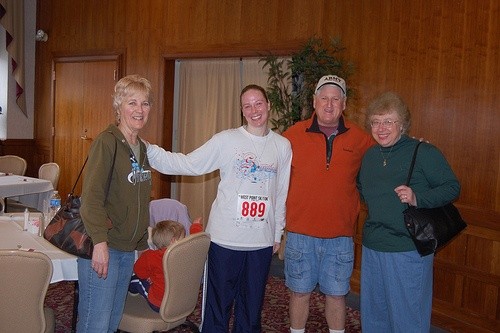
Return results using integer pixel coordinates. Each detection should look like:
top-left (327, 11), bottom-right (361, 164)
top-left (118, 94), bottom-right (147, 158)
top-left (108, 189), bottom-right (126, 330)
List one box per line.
top-left (38, 213), bottom-right (45, 237)
top-left (49, 190), bottom-right (61, 222)
top-left (23, 208), bottom-right (30, 231)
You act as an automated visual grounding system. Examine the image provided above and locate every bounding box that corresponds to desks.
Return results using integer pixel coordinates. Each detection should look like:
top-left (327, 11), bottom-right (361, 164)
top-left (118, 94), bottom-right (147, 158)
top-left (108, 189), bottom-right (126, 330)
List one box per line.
top-left (0, 212), bottom-right (80, 284)
top-left (0, 173), bottom-right (54, 214)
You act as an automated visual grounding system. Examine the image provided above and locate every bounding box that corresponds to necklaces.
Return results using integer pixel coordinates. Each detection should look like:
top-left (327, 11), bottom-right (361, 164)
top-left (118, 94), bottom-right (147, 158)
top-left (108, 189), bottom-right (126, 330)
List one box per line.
top-left (379, 147), bottom-right (392, 167)
top-left (251, 136), bottom-right (268, 161)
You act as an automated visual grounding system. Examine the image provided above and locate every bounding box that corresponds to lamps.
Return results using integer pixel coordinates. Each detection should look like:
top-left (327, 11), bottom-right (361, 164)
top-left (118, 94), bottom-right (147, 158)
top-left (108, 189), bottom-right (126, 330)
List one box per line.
top-left (36, 29), bottom-right (48, 42)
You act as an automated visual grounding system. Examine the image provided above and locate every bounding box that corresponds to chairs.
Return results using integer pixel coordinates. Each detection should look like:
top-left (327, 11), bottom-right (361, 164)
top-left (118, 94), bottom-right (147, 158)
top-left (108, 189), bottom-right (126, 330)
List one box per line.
top-left (0, 154), bottom-right (28, 176)
top-left (39, 162), bottom-right (60, 190)
top-left (0, 249), bottom-right (57, 333)
top-left (117, 232), bottom-right (211, 333)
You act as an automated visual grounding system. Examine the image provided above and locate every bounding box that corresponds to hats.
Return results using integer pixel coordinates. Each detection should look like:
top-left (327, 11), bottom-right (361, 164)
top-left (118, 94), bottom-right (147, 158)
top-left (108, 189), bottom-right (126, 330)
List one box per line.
top-left (315, 75), bottom-right (346, 96)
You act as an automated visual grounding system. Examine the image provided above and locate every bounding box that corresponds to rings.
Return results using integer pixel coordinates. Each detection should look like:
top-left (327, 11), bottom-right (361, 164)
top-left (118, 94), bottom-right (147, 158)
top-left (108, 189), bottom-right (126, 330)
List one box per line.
top-left (404, 195), bottom-right (407, 199)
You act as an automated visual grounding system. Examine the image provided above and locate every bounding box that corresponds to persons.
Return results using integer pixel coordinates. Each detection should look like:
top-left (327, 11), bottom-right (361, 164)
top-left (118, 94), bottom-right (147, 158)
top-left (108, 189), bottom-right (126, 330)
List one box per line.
top-left (75, 74), bottom-right (153, 333)
top-left (279, 74), bottom-right (430, 333)
top-left (128, 217), bottom-right (205, 313)
top-left (355, 92), bottom-right (460, 333)
top-left (138, 84), bottom-right (292, 333)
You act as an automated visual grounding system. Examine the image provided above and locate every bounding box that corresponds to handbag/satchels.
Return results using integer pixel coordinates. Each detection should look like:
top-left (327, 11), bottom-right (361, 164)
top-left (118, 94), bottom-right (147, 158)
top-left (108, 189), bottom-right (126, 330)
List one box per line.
top-left (43, 131), bottom-right (118, 260)
top-left (404, 141), bottom-right (467, 256)
